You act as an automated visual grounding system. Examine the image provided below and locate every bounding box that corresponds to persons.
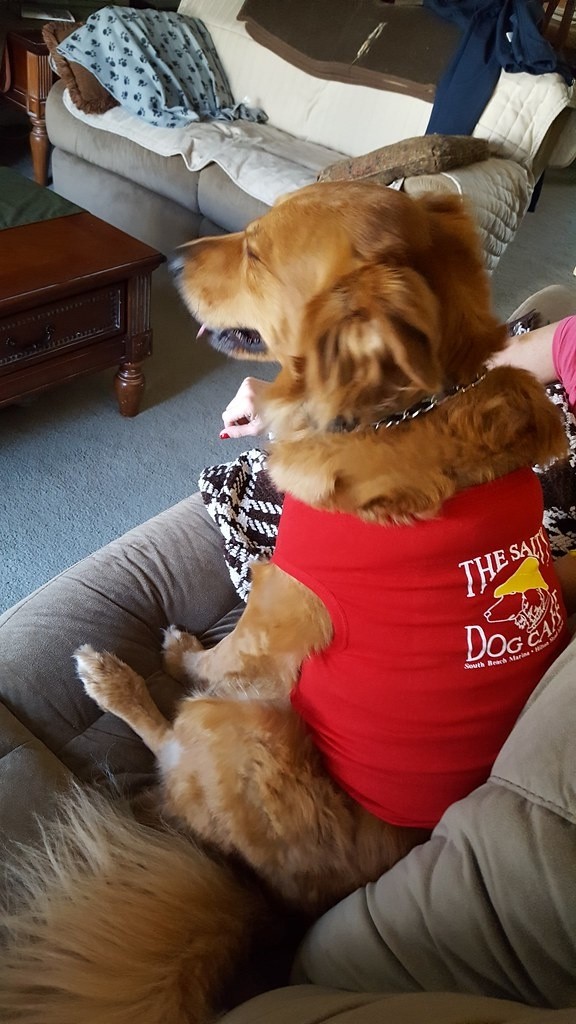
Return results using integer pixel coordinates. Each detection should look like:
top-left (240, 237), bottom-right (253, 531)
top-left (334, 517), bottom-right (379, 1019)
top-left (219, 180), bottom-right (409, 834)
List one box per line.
top-left (219, 313), bottom-right (575, 440)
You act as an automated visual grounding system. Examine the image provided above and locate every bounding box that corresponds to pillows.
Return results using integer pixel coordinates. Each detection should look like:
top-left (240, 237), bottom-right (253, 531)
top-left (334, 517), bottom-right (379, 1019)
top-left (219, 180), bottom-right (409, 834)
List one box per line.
top-left (315, 132), bottom-right (489, 187)
top-left (42, 18), bottom-right (123, 112)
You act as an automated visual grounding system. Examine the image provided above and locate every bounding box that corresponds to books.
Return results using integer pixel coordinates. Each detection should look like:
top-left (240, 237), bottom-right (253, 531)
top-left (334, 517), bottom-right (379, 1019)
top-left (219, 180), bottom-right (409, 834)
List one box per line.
top-left (21, 6), bottom-right (75, 23)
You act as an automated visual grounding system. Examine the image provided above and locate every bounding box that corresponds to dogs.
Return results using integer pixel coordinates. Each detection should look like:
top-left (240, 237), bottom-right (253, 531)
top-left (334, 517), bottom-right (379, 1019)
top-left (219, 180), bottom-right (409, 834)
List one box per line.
top-left (0, 178), bottom-right (569, 1023)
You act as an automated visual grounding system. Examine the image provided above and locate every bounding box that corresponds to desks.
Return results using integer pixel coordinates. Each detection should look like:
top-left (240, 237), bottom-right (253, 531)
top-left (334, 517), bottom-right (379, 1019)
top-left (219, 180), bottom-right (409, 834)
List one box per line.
top-left (0, 162), bottom-right (167, 418)
top-left (0, 0), bottom-right (157, 187)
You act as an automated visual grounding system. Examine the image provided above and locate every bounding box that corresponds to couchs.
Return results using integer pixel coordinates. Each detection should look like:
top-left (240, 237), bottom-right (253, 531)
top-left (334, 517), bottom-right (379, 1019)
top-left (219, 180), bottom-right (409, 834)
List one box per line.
top-left (44, 0), bottom-right (576, 288)
top-left (0, 280), bottom-right (576, 1024)
